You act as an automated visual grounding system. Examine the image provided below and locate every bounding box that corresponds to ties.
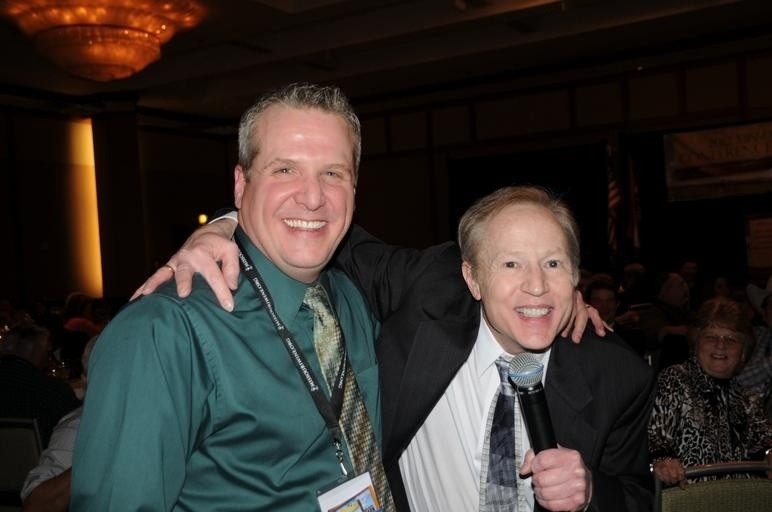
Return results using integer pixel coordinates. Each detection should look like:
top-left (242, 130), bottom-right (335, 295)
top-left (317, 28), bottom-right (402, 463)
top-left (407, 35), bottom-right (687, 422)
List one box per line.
top-left (301, 282), bottom-right (397, 512)
top-left (478, 353), bottom-right (536, 511)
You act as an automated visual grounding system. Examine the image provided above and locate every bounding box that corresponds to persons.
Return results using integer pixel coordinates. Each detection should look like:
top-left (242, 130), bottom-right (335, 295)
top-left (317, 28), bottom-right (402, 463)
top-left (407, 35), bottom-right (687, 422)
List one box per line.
top-left (0, 265), bottom-right (772, 512)
top-left (70, 76), bottom-right (624, 512)
top-left (129, 175), bottom-right (654, 512)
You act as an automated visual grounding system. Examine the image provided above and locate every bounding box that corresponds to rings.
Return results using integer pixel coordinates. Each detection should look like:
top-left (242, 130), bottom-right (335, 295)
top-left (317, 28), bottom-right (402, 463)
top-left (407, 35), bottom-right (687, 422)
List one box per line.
top-left (164, 263), bottom-right (176, 274)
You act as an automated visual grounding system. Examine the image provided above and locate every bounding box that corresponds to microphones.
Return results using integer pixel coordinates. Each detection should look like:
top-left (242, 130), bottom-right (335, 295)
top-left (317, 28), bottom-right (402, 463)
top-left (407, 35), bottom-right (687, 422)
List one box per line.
top-left (509, 352), bottom-right (558, 456)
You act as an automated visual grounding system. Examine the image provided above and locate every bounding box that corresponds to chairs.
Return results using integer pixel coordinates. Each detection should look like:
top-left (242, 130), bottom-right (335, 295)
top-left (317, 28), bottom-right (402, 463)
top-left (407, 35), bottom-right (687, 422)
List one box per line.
top-left (653, 460), bottom-right (772, 512)
top-left (0, 414), bottom-right (45, 493)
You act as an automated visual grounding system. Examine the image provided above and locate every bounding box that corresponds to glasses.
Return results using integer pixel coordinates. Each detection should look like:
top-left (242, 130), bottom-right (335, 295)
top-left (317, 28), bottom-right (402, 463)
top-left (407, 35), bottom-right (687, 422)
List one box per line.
top-left (701, 328), bottom-right (743, 344)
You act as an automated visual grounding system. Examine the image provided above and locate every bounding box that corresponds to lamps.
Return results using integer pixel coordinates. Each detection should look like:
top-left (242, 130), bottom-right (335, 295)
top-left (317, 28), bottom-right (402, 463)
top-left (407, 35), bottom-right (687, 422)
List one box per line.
top-left (6, 0), bottom-right (207, 83)
